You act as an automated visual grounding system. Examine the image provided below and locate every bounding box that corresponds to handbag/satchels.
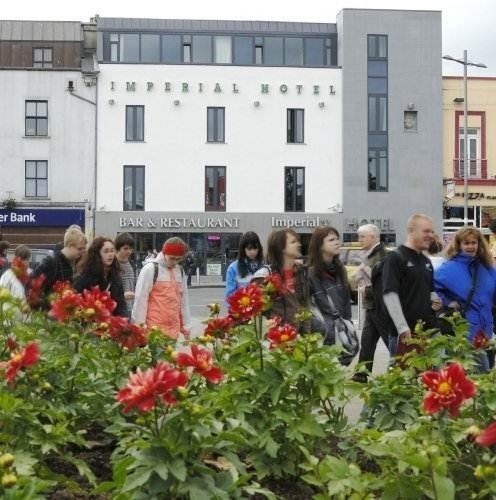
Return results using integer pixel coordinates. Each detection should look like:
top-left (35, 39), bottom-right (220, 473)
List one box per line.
top-left (334, 317), bottom-right (359, 364)
top-left (435, 305), bottom-right (465, 337)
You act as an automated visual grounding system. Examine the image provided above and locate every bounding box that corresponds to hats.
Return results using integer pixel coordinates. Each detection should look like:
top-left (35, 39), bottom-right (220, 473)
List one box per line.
top-left (164, 237), bottom-right (186, 257)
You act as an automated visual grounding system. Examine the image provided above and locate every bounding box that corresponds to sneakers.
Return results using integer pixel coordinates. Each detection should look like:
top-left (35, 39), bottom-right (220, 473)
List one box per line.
top-left (350, 376), bottom-right (366, 382)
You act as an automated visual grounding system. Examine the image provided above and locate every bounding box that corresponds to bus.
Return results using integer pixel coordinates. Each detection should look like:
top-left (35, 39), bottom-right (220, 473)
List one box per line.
top-left (5, 248), bottom-right (53, 273)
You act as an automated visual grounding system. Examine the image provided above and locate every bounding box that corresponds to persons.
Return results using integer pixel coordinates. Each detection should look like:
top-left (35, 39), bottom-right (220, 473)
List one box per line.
top-left (0, 224), bottom-right (197, 347)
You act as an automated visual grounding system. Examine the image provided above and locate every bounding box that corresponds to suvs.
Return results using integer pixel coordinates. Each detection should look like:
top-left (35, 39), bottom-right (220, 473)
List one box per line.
top-left (296, 239), bottom-right (399, 303)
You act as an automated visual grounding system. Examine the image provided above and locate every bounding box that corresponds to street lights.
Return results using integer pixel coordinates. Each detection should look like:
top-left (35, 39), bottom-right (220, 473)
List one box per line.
top-left (441, 50), bottom-right (487, 229)
